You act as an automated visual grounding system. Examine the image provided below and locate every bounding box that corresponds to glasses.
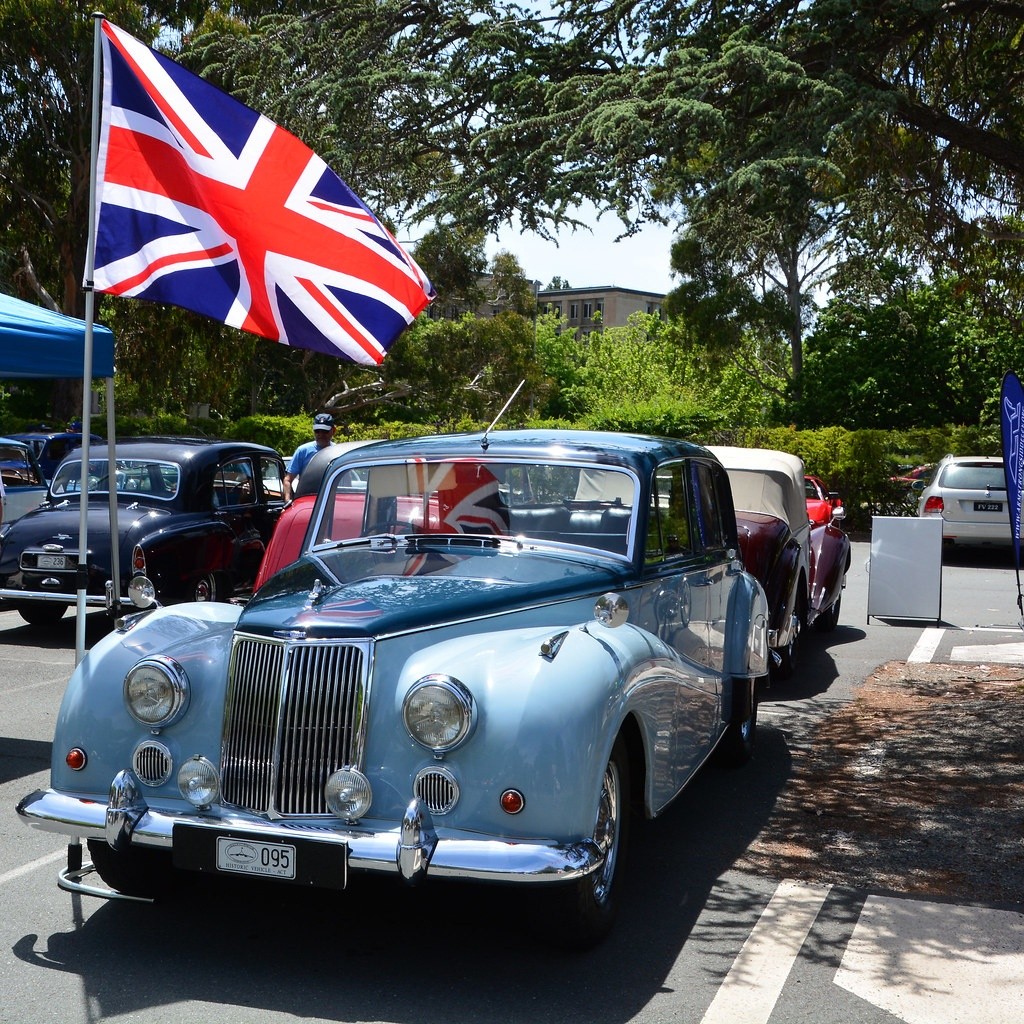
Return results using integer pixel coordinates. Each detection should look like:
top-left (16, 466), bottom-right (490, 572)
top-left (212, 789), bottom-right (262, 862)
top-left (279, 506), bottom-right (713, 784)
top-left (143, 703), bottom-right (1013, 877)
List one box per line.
top-left (314, 428), bottom-right (333, 434)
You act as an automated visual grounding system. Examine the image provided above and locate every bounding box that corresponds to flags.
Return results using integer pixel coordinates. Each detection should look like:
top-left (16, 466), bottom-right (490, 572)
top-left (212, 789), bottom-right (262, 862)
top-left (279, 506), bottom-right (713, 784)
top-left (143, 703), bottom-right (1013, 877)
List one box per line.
top-left (84, 20), bottom-right (432, 369)
top-left (388, 460), bottom-right (514, 575)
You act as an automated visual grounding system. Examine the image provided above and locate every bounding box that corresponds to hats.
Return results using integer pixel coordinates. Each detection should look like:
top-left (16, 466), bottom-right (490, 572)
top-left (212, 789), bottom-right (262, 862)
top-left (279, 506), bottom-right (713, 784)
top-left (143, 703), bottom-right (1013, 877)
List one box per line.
top-left (313, 413), bottom-right (334, 431)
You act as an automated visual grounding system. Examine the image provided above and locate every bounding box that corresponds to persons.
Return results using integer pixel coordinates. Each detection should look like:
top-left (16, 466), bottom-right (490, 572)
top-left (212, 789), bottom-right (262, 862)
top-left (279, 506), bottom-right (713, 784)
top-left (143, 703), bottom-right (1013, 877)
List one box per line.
top-left (283, 413), bottom-right (352, 503)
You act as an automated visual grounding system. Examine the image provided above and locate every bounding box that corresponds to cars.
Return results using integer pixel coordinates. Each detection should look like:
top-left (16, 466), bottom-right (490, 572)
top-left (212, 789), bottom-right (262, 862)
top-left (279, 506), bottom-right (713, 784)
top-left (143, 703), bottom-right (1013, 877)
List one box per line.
top-left (889, 464), bottom-right (938, 485)
top-left (0, 434), bottom-right (296, 625)
top-left (88, 463), bottom-right (253, 493)
top-left (0, 437), bottom-right (50, 524)
top-left (243, 457), bottom-right (368, 493)
top-left (251, 487), bottom-right (439, 592)
top-left (574, 446), bottom-right (852, 680)
top-left (15, 378), bottom-right (769, 944)
top-left (803, 475), bottom-right (843, 529)
top-left (5, 432), bottom-right (104, 479)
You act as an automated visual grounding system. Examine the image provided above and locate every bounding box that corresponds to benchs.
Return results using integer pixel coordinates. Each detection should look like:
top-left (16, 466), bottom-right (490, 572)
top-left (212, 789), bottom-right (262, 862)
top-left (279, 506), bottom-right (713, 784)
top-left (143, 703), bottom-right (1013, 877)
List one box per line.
top-left (511, 507), bottom-right (630, 553)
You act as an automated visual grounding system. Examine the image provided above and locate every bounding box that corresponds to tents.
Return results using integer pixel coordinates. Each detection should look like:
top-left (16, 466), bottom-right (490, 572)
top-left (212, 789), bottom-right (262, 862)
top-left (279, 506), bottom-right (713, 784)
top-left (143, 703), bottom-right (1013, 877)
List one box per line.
top-left (0, 293), bottom-right (114, 381)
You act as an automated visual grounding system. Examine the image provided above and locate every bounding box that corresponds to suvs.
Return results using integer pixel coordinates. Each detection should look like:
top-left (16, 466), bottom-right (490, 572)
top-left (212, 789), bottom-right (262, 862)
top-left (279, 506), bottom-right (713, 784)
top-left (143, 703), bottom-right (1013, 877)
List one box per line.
top-left (912, 454), bottom-right (1024, 539)
